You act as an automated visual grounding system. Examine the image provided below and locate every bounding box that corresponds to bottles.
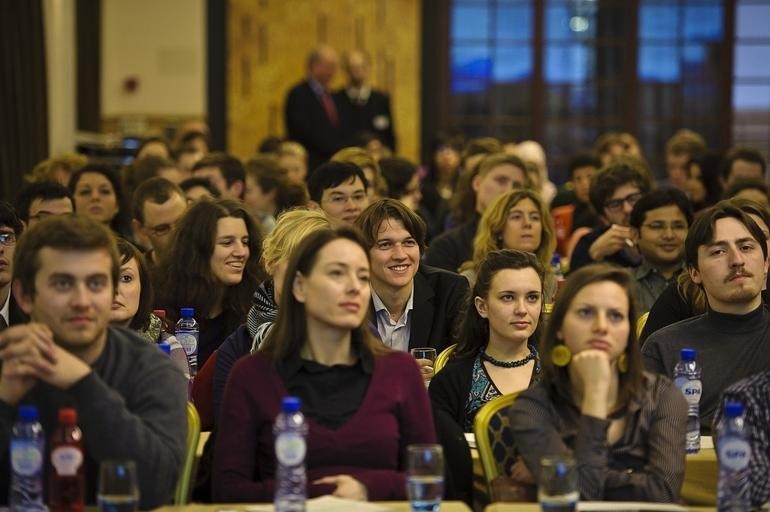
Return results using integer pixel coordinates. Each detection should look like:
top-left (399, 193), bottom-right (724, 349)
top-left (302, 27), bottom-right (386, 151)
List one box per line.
top-left (9, 404), bottom-right (85, 512)
top-left (548, 255), bottom-right (564, 281)
top-left (270, 396), bottom-right (309, 512)
top-left (716, 402), bottom-right (753, 512)
top-left (151, 307), bottom-right (200, 379)
top-left (671, 348), bottom-right (701, 456)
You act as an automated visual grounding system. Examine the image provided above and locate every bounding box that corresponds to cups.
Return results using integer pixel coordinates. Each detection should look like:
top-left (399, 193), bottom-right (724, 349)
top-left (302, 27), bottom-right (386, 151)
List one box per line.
top-left (97, 459), bottom-right (140, 511)
top-left (536, 455), bottom-right (580, 512)
top-left (411, 346), bottom-right (436, 385)
top-left (404, 443), bottom-right (445, 512)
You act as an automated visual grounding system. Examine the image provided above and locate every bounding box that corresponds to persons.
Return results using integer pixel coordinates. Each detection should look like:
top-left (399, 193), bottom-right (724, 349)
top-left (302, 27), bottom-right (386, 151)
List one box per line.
top-left (507, 261), bottom-right (690, 503)
top-left (211, 223), bottom-right (442, 505)
top-left (635, 200), bottom-right (770, 435)
top-left (215, 207), bottom-right (341, 434)
top-left (710, 363), bottom-right (770, 511)
top-left (426, 249), bottom-right (560, 503)
top-left (0, 214), bottom-right (190, 512)
top-left (1, 37), bottom-right (770, 378)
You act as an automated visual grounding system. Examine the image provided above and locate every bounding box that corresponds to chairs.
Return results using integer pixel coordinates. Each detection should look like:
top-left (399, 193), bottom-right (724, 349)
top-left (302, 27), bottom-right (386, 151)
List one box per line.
top-left (474, 394), bottom-right (539, 505)
top-left (433, 342), bottom-right (460, 376)
top-left (173, 401), bottom-right (202, 508)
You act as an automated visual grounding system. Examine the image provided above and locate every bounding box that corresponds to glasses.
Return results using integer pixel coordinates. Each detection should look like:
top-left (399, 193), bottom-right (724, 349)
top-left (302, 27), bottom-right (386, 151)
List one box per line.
top-left (323, 191), bottom-right (368, 205)
top-left (1, 231), bottom-right (20, 247)
top-left (603, 192), bottom-right (643, 212)
top-left (144, 224), bottom-right (171, 237)
top-left (28, 212), bottom-right (54, 220)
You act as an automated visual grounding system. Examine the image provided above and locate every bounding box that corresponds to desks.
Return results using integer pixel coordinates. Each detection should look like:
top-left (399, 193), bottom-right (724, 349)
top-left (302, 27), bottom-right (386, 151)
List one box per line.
top-left (468, 444), bottom-right (720, 507)
top-left (485, 501), bottom-right (716, 512)
top-left (153, 498), bottom-right (476, 512)
